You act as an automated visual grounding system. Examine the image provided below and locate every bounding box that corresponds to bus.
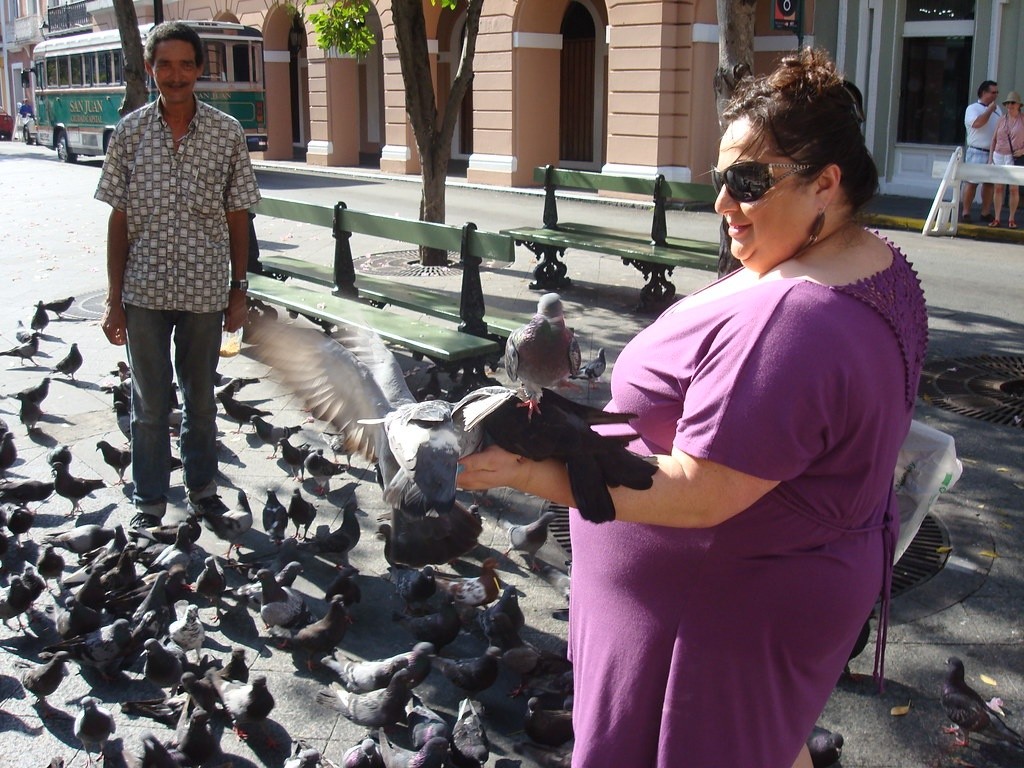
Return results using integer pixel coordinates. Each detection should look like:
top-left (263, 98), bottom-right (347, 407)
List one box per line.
top-left (21, 20), bottom-right (268, 163)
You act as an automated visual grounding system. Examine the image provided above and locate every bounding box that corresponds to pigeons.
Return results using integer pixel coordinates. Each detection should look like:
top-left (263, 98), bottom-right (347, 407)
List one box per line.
top-left (939, 657), bottom-right (1024, 751)
top-left (807, 727), bottom-right (844, 768)
top-left (0, 291), bottom-right (659, 762)
top-left (843, 608), bottom-right (877, 684)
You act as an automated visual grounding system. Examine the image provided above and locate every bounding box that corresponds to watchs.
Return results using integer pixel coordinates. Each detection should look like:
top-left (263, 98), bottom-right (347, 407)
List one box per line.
top-left (229, 280), bottom-right (251, 293)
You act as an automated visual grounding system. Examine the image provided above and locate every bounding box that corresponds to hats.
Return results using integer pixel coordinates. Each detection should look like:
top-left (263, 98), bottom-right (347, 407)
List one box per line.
top-left (1002, 92), bottom-right (1024, 108)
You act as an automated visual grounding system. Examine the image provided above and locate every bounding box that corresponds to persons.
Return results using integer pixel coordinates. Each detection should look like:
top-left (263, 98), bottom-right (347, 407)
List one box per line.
top-left (959, 80), bottom-right (1003, 227)
top-left (19, 98), bottom-right (34, 118)
top-left (985, 89), bottom-right (1024, 229)
top-left (452, 47), bottom-right (930, 768)
top-left (93, 19), bottom-right (263, 533)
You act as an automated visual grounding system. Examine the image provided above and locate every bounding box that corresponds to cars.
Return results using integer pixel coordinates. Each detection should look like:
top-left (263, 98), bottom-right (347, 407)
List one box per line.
top-left (0, 106), bottom-right (14, 141)
top-left (23, 113), bottom-right (41, 145)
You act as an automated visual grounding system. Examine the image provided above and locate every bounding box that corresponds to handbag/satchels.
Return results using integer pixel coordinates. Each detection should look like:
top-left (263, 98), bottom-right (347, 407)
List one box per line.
top-left (1013, 154), bottom-right (1024, 166)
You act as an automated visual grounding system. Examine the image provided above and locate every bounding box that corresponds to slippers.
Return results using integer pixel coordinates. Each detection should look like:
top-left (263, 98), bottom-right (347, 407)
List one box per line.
top-left (1008, 220), bottom-right (1017, 229)
top-left (989, 220), bottom-right (1000, 227)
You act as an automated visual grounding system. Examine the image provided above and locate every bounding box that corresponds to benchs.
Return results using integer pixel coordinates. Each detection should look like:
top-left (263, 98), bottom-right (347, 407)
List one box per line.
top-left (497, 162), bottom-right (721, 316)
top-left (229, 197), bottom-right (574, 404)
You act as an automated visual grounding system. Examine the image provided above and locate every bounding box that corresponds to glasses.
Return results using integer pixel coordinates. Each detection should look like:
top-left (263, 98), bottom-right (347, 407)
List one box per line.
top-left (1006, 101), bottom-right (1016, 105)
top-left (710, 162), bottom-right (814, 204)
top-left (990, 91), bottom-right (999, 94)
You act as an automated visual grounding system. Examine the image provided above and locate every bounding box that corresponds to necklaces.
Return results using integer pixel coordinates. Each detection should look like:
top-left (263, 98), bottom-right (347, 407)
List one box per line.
top-left (173, 132), bottom-right (189, 145)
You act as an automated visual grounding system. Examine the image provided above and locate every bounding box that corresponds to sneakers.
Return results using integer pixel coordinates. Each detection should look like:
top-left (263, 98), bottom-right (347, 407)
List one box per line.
top-left (131, 514), bottom-right (160, 527)
top-left (187, 494), bottom-right (229, 531)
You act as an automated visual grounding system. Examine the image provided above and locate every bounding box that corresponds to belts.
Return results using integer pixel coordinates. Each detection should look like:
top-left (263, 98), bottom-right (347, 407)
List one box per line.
top-left (972, 147), bottom-right (989, 152)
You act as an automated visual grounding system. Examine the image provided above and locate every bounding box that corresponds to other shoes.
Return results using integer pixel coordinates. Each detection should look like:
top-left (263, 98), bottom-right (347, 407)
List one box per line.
top-left (962, 213), bottom-right (972, 224)
top-left (979, 213), bottom-right (996, 222)
